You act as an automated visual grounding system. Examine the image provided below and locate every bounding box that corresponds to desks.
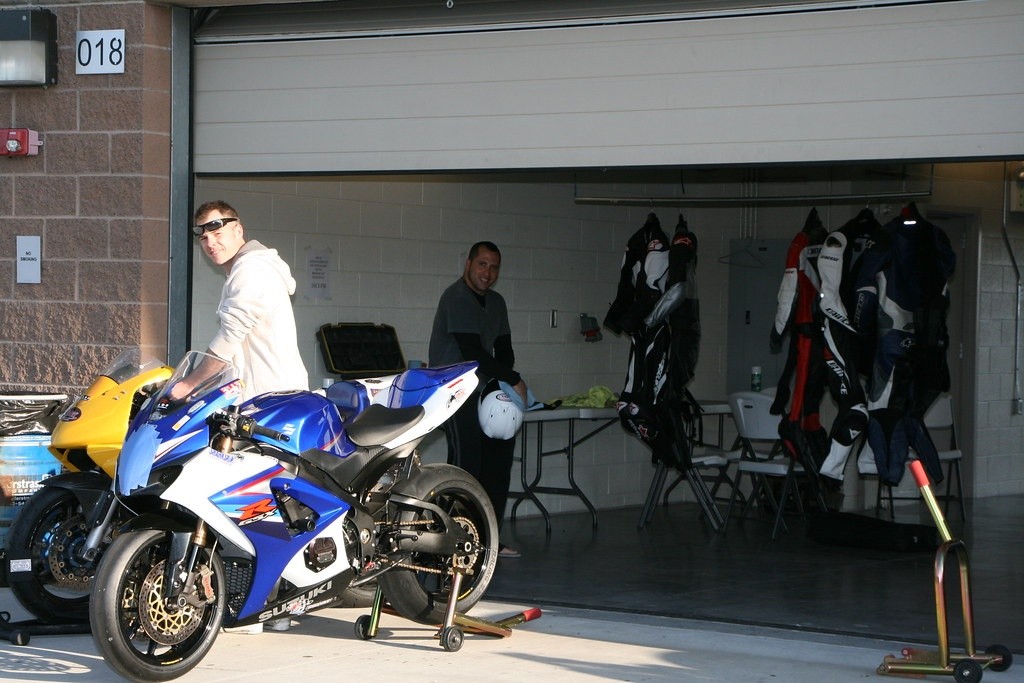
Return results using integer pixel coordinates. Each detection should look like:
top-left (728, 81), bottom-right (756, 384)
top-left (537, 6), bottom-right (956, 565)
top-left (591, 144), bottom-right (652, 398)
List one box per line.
top-left (507, 400), bottom-right (746, 540)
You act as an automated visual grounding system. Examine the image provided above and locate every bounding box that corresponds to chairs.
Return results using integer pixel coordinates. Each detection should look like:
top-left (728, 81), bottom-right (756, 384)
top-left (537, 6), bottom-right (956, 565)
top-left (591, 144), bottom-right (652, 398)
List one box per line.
top-left (875, 394), bottom-right (968, 522)
top-left (637, 391), bottom-right (827, 539)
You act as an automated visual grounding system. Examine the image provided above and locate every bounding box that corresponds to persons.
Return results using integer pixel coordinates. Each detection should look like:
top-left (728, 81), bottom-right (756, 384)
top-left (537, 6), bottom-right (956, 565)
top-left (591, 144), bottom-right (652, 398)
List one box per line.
top-left (429, 241), bottom-right (527, 558)
top-left (168, 201), bottom-right (310, 633)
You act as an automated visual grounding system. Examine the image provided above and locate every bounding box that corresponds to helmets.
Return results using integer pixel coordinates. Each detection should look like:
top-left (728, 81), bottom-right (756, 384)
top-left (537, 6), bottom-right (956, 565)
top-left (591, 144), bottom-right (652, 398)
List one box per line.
top-left (477, 376), bottom-right (523, 440)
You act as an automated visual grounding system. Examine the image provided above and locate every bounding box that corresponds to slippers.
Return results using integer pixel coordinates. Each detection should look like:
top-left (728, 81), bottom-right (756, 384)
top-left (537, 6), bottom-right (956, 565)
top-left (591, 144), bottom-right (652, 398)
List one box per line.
top-left (496, 545), bottom-right (521, 557)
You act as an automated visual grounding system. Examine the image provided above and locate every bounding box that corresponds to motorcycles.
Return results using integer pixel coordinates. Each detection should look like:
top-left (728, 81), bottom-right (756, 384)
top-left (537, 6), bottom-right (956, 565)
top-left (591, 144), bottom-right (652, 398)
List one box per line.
top-left (89, 352), bottom-right (502, 681)
top-left (1, 351), bottom-right (178, 628)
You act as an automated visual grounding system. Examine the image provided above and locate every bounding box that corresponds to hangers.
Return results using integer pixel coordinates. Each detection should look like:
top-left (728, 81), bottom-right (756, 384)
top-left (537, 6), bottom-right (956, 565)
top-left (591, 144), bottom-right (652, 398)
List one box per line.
top-left (854, 200), bottom-right (882, 227)
top-left (673, 201), bottom-right (687, 228)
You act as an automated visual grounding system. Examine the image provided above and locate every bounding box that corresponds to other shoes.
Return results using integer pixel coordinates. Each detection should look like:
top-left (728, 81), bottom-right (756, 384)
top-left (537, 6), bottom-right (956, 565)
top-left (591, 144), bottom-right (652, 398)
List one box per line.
top-left (216, 618), bottom-right (291, 634)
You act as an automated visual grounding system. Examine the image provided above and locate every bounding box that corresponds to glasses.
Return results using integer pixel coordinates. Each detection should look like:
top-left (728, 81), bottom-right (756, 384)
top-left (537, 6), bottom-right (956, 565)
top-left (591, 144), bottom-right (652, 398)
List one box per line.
top-left (191, 218), bottom-right (237, 236)
